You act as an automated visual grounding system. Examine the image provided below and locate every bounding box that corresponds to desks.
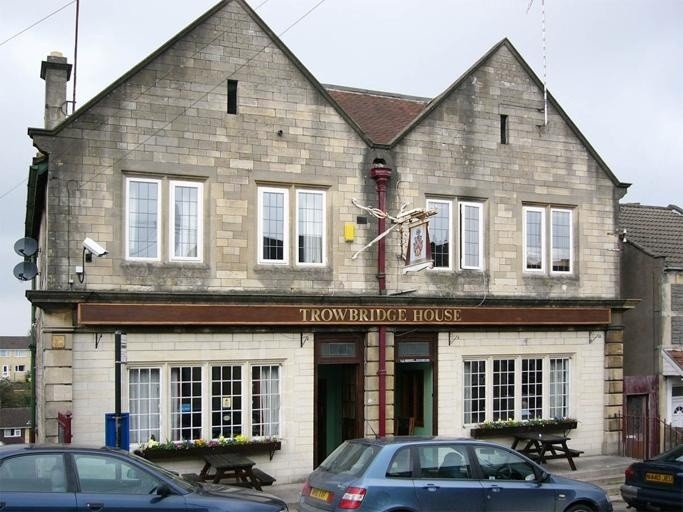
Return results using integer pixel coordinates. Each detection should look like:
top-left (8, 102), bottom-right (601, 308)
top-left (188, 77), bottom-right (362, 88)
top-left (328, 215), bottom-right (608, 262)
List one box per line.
top-left (199, 453), bottom-right (264, 493)
top-left (511, 432), bottom-right (577, 470)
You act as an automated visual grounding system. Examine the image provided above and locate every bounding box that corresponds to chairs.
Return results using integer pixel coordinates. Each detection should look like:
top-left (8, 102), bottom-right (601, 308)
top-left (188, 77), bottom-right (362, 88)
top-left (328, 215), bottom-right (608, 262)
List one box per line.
top-left (50, 465), bottom-right (68, 492)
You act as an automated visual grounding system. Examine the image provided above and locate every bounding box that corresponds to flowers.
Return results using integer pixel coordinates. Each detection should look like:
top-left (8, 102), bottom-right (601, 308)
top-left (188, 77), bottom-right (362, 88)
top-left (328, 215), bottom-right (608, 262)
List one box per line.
top-left (478, 416), bottom-right (576, 432)
top-left (139, 435), bottom-right (278, 455)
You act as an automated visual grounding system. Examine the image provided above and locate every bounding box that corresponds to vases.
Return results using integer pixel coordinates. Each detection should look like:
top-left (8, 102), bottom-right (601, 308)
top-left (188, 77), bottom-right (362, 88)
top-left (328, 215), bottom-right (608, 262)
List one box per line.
top-left (144, 442), bottom-right (281, 462)
top-left (470, 422), bottom-right (577, 439)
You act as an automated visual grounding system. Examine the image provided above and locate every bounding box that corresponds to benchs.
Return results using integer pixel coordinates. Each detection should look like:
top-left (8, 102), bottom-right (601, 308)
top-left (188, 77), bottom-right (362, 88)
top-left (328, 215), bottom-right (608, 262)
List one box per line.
top-left (540, 445), bottom-right (584, 457)
top-left (516, 449), bottom-right (538, 461)
top-left (182, 472), bottom-right (207, 483)
top-left (253, 468), bottom-right (276, 486)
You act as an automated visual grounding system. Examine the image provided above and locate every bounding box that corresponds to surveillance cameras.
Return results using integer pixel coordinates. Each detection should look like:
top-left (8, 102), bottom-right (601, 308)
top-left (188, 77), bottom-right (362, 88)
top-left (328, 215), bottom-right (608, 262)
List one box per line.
top-left (82, 237), bottom-right (109, 257)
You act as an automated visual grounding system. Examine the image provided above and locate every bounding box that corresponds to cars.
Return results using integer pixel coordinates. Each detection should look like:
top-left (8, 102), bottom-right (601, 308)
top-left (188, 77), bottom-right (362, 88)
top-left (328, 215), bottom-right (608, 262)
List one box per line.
top-left (619, 443), bottom-right (682, 512)
top-left (298, 437), bottom-right (615, 512)
top-left (0, 444), bottom-right (291, 511)
